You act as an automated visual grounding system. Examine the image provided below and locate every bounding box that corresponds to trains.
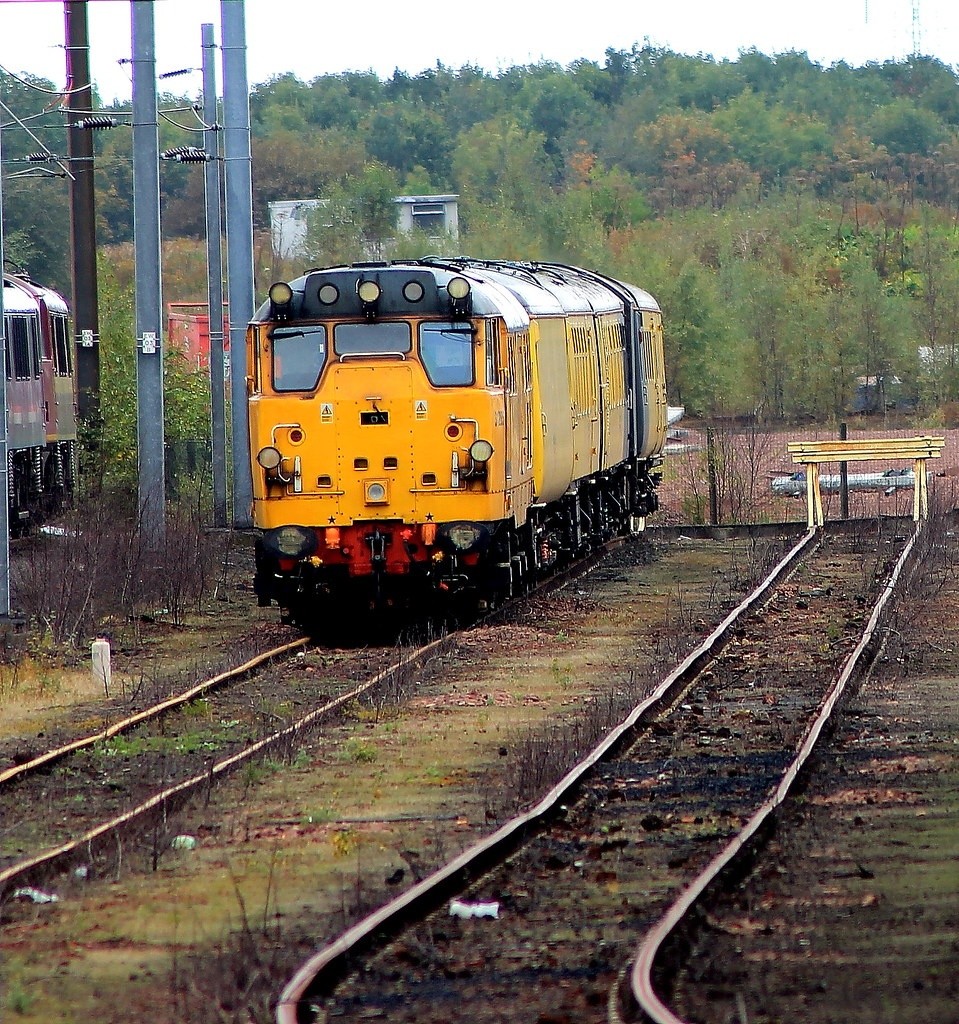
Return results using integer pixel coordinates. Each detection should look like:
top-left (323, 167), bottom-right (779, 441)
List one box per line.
top-left (246, 254), bottom-right (669, 642)
top-left (3, 257), bottom-right (82, 539)
